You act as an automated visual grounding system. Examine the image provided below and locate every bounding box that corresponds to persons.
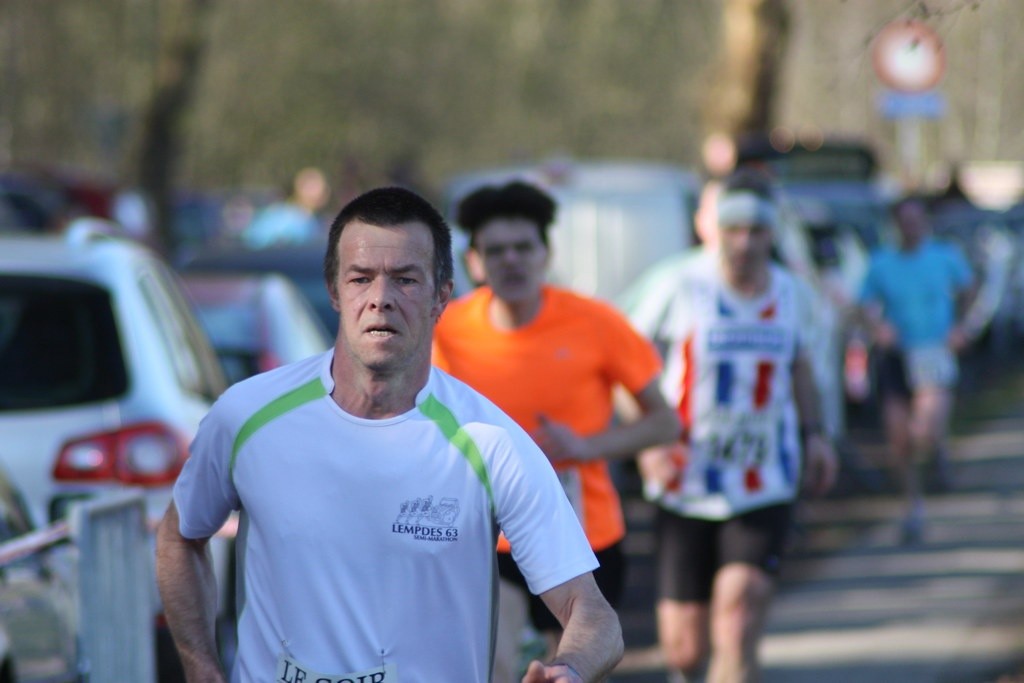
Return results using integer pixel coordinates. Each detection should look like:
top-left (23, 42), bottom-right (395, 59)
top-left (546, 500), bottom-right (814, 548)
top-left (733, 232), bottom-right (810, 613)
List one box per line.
top-left (611, 173), bottom-right (835, 683)
top-left (428, 181), bottom-right (680, 679)
top-left (247, 164), bottom-right (331, 249)
top-left (155, 185), bottom-right (624, 683)
top-left (859, 197), bottom-right (977, 545)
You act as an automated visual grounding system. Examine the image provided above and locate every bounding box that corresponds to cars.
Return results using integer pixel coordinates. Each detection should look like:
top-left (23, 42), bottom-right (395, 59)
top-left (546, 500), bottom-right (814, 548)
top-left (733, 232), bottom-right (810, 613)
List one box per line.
top-left (1, 163), bottom-right (1024, 683)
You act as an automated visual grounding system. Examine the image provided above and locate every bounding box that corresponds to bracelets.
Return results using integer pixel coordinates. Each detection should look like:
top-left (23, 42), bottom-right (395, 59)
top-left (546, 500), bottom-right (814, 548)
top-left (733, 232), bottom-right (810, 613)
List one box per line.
top-left (801, 423), bottom-right (821, 436)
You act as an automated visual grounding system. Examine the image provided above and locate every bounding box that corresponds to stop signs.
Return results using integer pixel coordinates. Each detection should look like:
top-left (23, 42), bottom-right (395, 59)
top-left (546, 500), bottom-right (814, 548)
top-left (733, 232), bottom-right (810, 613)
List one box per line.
top-left (872, 17), bottom-right (944, 92)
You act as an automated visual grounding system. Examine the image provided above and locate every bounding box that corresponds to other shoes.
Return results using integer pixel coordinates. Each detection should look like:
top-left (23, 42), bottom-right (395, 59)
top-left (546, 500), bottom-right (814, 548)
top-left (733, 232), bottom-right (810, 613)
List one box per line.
top-left (901, 503), bottom-right (923, 542)
